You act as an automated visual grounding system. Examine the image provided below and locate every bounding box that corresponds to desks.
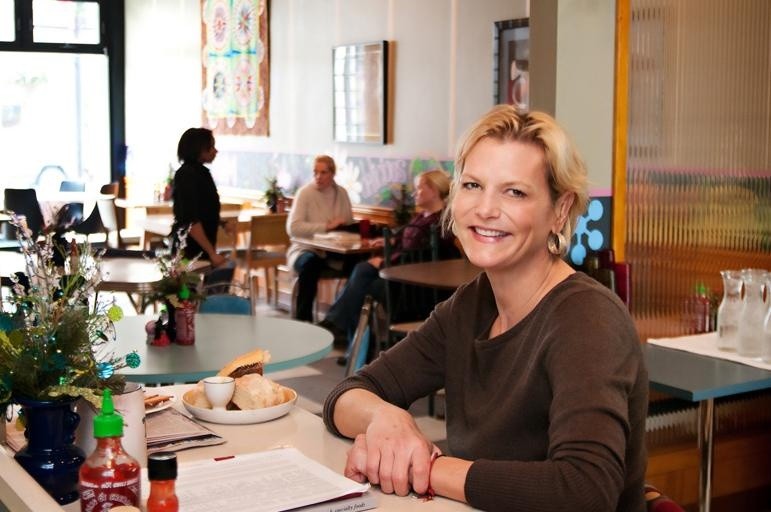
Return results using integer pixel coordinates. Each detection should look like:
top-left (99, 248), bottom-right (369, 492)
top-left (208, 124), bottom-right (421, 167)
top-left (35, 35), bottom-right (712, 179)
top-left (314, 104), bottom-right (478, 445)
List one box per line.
top-left (0, 192), bottom-right (771, 512)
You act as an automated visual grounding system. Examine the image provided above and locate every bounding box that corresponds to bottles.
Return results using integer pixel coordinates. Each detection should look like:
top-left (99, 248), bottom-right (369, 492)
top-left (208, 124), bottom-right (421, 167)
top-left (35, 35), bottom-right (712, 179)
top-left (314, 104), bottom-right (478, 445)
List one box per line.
top-left (152, 177), bottom-right (172, 203)
top-left (71, 238), bottom-right (80, 273)
top-left (144, 452), bottom-right (180, 512)
top-left (78, 389), bottom-right (141, 512)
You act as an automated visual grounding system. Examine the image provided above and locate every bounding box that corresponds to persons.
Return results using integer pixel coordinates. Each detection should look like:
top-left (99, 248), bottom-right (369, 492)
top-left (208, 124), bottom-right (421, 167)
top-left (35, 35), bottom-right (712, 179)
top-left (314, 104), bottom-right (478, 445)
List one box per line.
top-left (324, 102), bottom-right (649, 512)
top-left (172, 128), bottom-right (228, 268)
top-left (318, 171), bottom-right (463, 351)
top-left (286, 156), bottom-right (362, 325)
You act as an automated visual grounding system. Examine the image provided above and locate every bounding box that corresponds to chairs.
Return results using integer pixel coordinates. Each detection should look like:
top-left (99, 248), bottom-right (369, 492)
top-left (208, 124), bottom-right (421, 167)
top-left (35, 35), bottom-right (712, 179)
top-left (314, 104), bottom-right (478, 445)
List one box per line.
top-left (60, 182), bottom-right (85, 192)
top-left (5, 188), bottom-right (45, 238)
top-left (101, 181), bottom-right (119, 197)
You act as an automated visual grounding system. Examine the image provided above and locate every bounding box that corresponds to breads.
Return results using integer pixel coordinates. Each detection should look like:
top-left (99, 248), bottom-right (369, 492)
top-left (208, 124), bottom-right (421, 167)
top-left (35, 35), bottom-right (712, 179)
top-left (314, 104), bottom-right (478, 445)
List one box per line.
top-left (182, 349), bottom-right (294, 411)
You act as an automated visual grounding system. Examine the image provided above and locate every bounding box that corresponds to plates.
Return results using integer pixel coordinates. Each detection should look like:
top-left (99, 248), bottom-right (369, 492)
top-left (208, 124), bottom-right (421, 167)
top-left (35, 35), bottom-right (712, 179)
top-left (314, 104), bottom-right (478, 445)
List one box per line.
top-left (181, 385), bottom-right (298, 425)
top-left (142, 387), bottom-right (176, 414)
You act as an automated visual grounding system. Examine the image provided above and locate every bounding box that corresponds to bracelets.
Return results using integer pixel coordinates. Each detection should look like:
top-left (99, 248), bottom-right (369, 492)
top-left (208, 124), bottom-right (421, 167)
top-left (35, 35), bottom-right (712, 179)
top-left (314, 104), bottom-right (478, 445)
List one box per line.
top-left (418, 453), bottom-right (446, 503)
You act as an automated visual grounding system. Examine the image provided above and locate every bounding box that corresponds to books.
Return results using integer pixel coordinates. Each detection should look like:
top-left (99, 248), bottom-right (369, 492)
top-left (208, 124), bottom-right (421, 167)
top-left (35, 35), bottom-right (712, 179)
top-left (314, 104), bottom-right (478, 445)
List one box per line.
top-left (279, 491), bottom-right (380, 512)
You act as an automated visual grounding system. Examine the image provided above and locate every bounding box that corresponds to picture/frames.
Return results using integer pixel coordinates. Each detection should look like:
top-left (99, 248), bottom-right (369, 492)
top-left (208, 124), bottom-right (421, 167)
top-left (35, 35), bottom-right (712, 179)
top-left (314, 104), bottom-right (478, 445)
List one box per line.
top-left (493, 18), bottom-right (530, 108)
top-left (332, 41), bottom-right (388, 146)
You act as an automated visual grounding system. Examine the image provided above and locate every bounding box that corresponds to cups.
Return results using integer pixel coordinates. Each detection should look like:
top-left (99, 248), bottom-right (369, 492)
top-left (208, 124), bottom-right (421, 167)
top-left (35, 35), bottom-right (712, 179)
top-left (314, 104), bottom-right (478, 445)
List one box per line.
top-left (204, 376), bottom-right (236, 411)
top-left (358, 220), bottom-right (369, 247)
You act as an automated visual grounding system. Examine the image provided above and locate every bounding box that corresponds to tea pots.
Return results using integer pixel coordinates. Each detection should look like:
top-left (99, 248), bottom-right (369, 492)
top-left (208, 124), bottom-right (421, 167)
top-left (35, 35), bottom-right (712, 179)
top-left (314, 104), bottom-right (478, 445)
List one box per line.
top-left (716, 268), bottom-right (771, 363)
